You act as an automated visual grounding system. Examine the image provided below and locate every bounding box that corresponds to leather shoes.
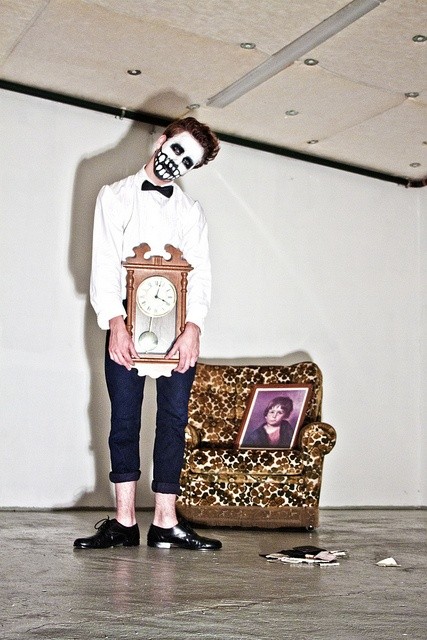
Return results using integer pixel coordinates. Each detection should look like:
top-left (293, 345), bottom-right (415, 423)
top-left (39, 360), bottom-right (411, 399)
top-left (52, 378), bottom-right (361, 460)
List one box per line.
top-left (74, 518), bottom-right (140, 550)
top-left (147, 521), bottom-right (222, 551)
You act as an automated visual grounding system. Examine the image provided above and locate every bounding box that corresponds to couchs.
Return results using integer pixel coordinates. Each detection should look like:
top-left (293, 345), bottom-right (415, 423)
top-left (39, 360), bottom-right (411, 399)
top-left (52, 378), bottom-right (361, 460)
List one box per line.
top-left (174, 360), bottom-right (337, 532)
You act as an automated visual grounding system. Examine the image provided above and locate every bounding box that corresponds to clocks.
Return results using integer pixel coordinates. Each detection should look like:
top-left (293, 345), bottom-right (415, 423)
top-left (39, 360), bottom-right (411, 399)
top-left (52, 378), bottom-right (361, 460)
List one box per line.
top-left (121, 241), bottom-right (194, 364)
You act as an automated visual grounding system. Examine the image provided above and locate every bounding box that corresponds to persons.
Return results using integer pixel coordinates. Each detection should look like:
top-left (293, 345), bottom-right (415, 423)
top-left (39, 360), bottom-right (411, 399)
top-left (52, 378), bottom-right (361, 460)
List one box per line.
top-left (241, 396), bottom-right (294, 448)
top-left (72, 116), bottom-right (221, 552)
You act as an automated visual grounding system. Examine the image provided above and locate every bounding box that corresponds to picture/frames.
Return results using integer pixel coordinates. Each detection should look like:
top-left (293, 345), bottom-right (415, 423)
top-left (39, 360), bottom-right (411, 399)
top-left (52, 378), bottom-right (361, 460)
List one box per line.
top-left (235, 382), bottom-right (314, 452)
